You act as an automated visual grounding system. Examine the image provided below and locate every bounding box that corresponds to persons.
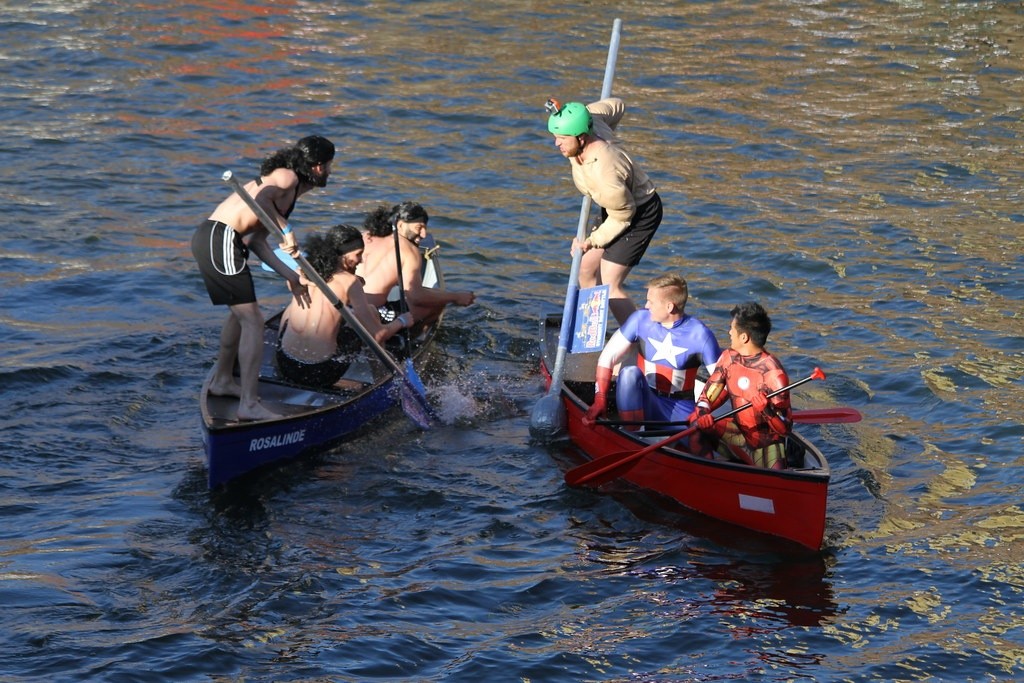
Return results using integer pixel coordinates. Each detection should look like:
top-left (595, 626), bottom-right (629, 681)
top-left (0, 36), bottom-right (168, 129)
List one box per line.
top-left (276, 223), bottom-right (414, 387)
top-left (191, 135), bottom-right (335, 421)
top-left (689, 303), bottom-right (793, 469)
top-left (548, 97), bottom-right (664, 324)
top-left (354, 201), bottom-right (475, 342)
top-left (581, 273), bottom-right (729, 460)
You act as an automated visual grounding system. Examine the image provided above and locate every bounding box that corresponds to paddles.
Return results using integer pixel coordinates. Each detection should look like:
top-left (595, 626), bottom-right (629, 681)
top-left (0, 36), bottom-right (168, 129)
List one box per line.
top-left (258, 243), bottom-right (452, 293)
top-left (590, 406), bottom-right (862, 426)
top-left (565, 366), bottom-right (824, 488)
top-left (221, 170), bottom-right (434, 430)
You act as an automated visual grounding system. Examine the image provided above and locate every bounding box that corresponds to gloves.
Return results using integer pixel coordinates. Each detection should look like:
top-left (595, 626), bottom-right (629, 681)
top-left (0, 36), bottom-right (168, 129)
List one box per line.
top-left (751, 392), bottom-right (768, 412)
top-left (698, 414), bottom-right (715, 431)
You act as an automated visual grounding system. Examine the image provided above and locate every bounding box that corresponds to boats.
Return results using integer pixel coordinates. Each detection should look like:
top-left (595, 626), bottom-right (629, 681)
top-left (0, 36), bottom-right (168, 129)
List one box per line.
top-left (197, 233), bottom-right (446, 490)
top-left (534, 302), bottom-right (830, 552)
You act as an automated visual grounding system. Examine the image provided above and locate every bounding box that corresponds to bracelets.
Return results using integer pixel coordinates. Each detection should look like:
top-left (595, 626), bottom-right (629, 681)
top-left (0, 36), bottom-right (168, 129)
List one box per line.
top-left (397, 316), bottom-right (406, 327)
top-left (282, 224), bottom-right (292, 234)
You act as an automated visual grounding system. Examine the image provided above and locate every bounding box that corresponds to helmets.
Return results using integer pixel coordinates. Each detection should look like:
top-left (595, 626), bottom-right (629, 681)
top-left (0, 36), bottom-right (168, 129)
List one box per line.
top-left (545, 98), bottom-right (593, 137)
